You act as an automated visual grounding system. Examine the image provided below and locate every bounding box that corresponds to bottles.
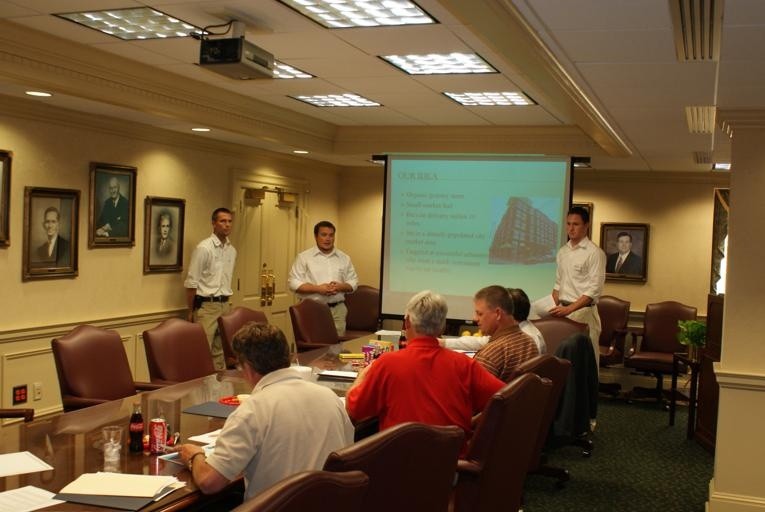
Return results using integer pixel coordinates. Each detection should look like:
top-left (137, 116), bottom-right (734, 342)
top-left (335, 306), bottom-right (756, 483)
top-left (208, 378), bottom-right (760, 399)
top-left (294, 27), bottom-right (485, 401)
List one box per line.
top-left (149, 418), bottom-right (166, 455)
top-left (399, 330), bottom-right (407, 350)
top-left (129, 401), bottom-right (145, 457)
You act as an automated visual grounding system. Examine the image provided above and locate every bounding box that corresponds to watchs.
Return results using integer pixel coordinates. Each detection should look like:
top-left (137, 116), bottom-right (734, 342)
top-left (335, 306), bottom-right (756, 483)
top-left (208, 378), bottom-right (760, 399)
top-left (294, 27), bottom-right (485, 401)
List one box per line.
top-left (186, 451), bottom-right (205, 472)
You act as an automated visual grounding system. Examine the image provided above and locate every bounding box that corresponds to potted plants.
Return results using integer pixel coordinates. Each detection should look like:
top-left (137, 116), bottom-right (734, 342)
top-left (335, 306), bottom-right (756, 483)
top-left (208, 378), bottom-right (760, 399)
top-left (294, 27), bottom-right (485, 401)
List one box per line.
top-left (675, 316), bottom-right (708, 360)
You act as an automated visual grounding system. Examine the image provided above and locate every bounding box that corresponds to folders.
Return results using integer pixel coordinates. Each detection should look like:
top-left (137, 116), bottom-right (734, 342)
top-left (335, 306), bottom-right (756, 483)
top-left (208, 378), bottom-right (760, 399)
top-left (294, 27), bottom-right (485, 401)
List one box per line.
top-left (51, 486), bottom-right (174, 512)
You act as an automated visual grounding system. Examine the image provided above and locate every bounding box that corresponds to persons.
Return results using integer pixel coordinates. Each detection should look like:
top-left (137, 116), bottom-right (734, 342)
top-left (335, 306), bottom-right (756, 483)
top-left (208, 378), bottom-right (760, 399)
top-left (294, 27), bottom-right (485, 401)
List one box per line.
top-left (471, 285), bottom-right (540, 383)
top-left (606, 232), bottom-right (644, 274)
top-left (183, 208), bottom-right (238, 370)
top-left (344, 290), bottom-right (507, 459)
top-left (287, 220), bottom-right (359, 337)
top-left (155, 212), bottom-right (175, 259)
top-left (549, 207), bottom-right (607, 434)
top-left (509, 288), bottom-right (548, 356)
top-left (37, 206), bottom-right (70, 267)
top-left (95, 176), bottom-right (130, 237)
top-left (166, 321), bottom-right (355, 504)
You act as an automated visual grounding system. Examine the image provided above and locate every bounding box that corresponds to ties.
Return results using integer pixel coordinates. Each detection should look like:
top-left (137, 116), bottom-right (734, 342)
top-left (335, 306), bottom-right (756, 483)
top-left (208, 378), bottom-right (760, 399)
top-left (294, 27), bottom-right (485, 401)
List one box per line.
top-left (616, 254), bottom-right (623, 271)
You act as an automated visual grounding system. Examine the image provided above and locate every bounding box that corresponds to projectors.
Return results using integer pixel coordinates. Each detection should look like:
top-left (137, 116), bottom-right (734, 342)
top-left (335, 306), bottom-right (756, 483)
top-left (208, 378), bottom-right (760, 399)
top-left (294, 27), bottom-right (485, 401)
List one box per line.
top-left (199, 37), bottom-right (274, 81)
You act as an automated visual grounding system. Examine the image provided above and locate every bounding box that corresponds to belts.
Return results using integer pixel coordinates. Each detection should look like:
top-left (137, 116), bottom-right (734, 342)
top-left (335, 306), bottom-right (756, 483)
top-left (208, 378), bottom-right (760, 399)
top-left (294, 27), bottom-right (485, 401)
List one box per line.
top-left (200, 295), bottom-right (231, 304)
top-left (558, 299), bottom-right (597, 309)
top-left (327, 301), bottom-right (345, 306)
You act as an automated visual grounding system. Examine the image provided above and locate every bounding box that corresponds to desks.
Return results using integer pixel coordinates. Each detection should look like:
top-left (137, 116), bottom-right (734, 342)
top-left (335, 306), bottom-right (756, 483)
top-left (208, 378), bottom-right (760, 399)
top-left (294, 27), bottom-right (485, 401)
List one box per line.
top-left (0, 330), bottom-right (494, 512)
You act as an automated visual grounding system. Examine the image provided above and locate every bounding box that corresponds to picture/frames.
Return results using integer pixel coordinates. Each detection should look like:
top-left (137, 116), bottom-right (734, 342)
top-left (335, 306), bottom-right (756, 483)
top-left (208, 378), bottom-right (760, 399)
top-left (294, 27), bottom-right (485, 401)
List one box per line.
top-left (142, 194), bottom-right (188, 274)
top-left (21, 186), bottom-right (81, 284)
top-left (599, 221), bottom-right (652, 286)
top-left (0, 147), bottom-right (13, 250)
top-left (85, 159), bottom-right (139, 249)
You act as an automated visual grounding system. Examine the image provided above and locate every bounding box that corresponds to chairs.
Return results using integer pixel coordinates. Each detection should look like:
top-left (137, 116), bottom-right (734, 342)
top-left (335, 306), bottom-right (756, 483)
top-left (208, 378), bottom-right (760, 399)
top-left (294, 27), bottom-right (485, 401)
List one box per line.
top-left (218, 308), bottom-right (269, 369)
top-left (289, 297), bottom-right (350, 351)
top-left (224, 468), bottom-right (372, 512)
top-left (51, 323), bottom-right (168, 413)
top-left (319, 419), bottom-right (467, 512)
top-left (554, 332), bottom-right (602, 460)
top-left (502, 353), bottom-right (572, 493)
top-left (594, 294), bottom-right (632, 400)
top-left (143, 315), bottom-right (223, 384)
top-left (458, 373), bottom-right (555, 512)
top-left (344, 285), bottom-right (381, 338)
top-left (625, 301), bottom-right (700, 409)
top-left (529, 314), bottom-right (588, 351)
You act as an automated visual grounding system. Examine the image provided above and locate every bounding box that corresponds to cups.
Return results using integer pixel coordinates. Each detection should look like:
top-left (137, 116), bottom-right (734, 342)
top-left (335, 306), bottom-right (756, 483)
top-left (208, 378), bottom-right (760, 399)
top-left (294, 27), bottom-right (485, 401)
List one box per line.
top-left (102, 426), bottom-right (123, 462)
